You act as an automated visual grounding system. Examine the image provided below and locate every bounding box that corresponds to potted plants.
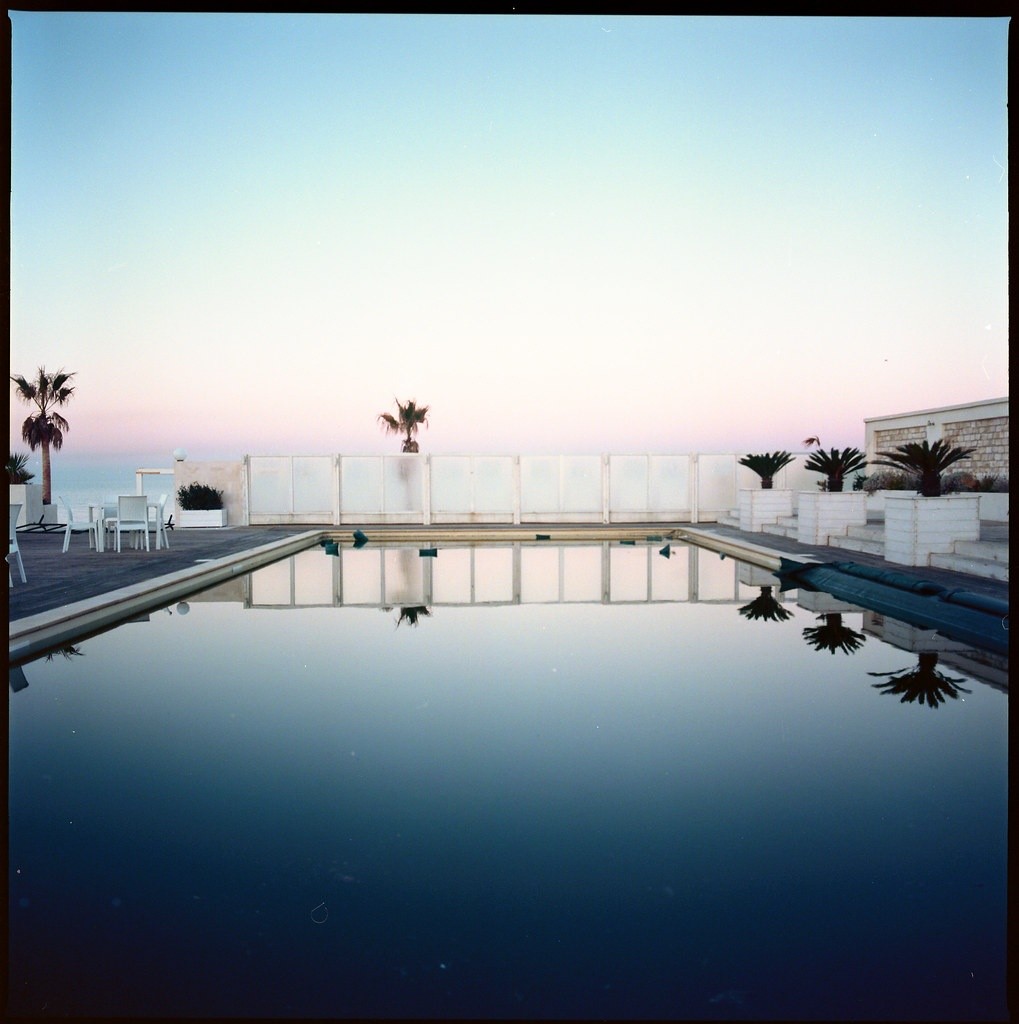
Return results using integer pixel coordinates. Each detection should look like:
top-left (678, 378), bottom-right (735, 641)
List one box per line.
top-left (737, 439), bottom-right (980, 566)
top-left (179, 479), bottom-right (227, 527)
top-left (736, 559), bottom-right (976, 709)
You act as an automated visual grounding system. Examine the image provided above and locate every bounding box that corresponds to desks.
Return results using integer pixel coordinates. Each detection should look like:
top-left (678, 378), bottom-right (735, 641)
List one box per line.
top-left (87, 502), bottom-right (161, 553)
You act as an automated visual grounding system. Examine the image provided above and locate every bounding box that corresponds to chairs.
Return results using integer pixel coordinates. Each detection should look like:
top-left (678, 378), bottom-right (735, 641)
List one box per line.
top-left (57, 493), bottom-right (170, 553)
top-left (4, 503), bottom-right (28, 588)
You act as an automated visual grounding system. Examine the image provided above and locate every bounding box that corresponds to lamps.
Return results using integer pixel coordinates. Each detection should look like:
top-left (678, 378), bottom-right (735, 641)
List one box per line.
top-left (172, 447), bottom-right (189, 462)
top-left (176, 601), bottom-right (192, 616)
